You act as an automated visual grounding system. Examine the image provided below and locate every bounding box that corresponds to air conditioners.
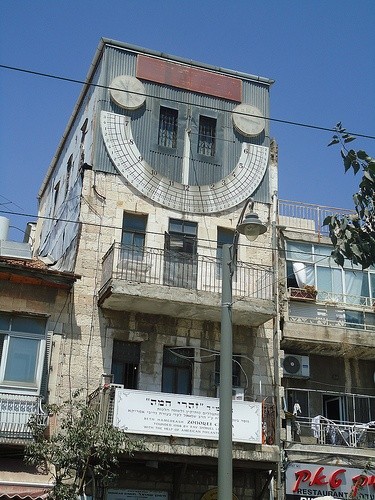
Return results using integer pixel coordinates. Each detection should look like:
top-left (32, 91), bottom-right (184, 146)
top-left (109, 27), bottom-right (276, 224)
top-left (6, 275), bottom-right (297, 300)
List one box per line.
top-left (280, 354), bottom-right (311, 380)
top-left (215, 385), bottom-right (244, 400)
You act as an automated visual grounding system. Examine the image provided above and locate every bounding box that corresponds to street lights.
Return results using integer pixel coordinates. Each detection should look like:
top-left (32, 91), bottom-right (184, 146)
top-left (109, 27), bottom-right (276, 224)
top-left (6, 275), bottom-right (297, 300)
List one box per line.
top-left (217, 197), bottom-right (268, 500)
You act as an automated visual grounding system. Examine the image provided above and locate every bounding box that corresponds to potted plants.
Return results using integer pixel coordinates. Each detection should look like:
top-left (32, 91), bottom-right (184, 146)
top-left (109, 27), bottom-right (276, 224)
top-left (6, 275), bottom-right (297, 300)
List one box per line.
top-left (291, 284), bottom-right (318, 301)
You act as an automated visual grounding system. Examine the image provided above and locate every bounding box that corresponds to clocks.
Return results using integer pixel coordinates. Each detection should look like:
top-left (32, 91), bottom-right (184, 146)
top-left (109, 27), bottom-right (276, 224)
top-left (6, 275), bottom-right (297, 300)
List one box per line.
top-left (233, 104), bottom-right (265, 136)
top-left (110, 75), bottom-right (146, 110)
